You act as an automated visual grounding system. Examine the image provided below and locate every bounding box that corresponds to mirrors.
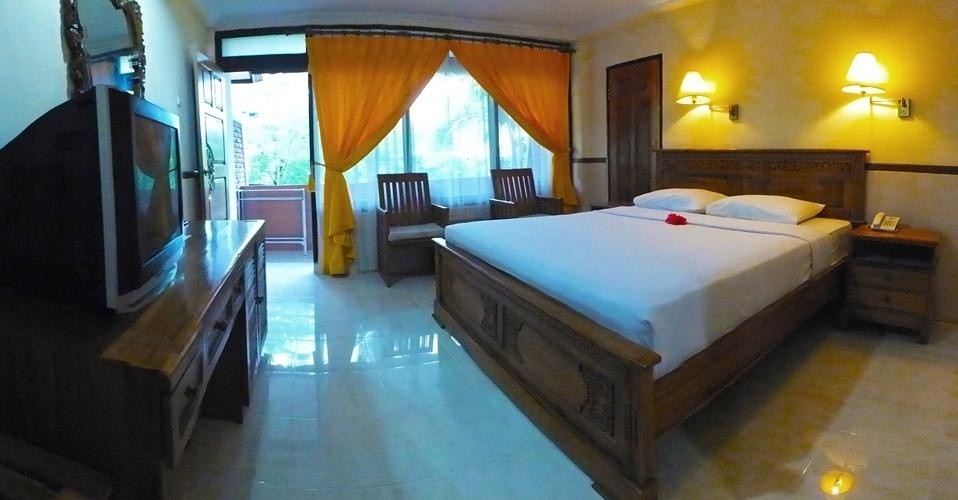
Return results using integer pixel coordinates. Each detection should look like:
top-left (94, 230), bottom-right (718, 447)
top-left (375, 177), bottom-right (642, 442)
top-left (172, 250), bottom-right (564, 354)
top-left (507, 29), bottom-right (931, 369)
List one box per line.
top-left (59, 0), bottom-right (147, 99)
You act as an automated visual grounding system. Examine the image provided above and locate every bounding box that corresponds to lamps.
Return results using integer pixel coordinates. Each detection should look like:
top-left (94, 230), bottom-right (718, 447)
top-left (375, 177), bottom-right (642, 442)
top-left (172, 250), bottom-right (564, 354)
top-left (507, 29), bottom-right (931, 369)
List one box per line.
top-left (673, 69), bottom-right (738, 122)
top-left (840, 52), bottom-right (910, 118)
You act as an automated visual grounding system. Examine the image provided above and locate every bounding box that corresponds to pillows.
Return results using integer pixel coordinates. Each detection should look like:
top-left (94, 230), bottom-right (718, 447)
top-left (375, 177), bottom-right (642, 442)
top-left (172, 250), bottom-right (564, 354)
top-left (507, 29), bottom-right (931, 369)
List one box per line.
top-left (630, 186), bottom-right (828, 226)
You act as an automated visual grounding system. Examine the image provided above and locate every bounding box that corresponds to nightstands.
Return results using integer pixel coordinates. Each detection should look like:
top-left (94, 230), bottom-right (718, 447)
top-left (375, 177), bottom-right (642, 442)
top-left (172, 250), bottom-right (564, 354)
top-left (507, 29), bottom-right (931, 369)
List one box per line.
top-left (836, 225), bottom-right (945, 346)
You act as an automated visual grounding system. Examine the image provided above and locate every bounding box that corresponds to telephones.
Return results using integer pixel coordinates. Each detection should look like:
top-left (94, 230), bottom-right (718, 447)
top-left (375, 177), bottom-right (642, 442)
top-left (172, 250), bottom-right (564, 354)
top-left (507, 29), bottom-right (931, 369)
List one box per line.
top-left (870, 212), bottom-right (901, 232)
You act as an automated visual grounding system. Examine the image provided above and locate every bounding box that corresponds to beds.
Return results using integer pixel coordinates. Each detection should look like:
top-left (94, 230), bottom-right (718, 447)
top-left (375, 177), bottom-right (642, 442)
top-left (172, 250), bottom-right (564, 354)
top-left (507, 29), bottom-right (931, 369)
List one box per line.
top-left (428, 146), bottom-right (872, 500)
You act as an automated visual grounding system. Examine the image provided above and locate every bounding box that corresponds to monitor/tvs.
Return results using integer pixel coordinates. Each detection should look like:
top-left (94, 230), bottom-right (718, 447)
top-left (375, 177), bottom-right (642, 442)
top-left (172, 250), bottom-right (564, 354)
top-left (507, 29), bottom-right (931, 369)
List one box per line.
top-left (0, 84), bottom-right (184, 313)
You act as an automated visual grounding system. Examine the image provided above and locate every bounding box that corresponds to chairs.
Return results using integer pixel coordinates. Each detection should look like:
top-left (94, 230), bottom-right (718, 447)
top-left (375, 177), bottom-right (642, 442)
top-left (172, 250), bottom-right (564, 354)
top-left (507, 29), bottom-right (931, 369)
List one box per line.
top-left (489, 168), bottom-right (563, 223)
top-left (375, 172), bottom-right (449, 287)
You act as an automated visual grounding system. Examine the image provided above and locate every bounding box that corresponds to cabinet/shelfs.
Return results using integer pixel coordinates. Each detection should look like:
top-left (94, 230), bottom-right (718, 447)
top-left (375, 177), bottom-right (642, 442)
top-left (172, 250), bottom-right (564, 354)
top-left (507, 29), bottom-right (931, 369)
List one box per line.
top-left (1, 219), bottom-right (270, 500)
